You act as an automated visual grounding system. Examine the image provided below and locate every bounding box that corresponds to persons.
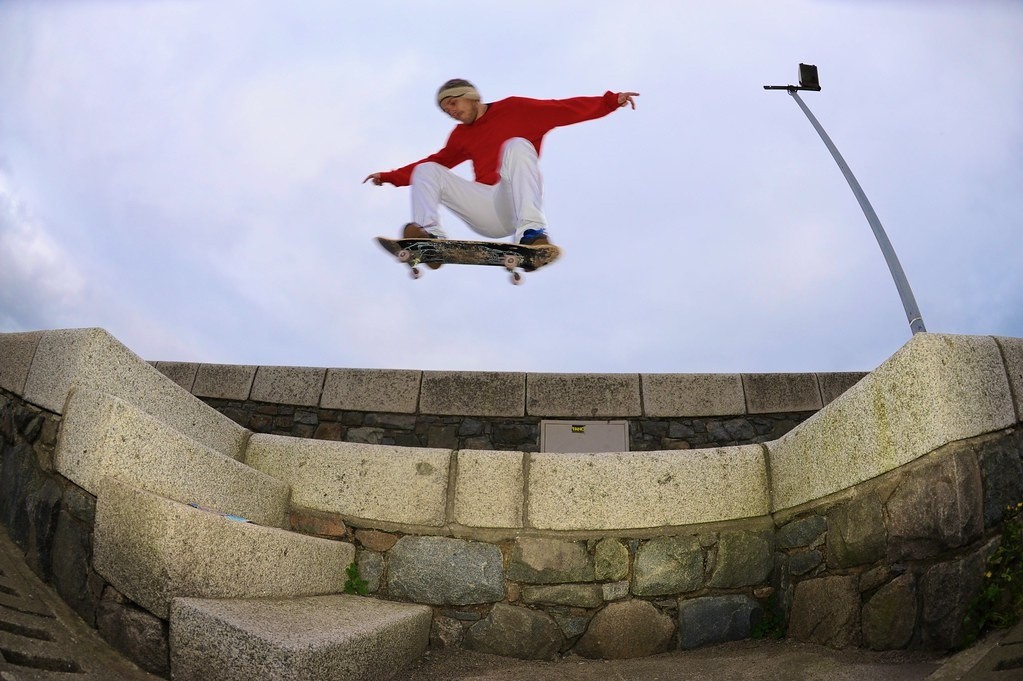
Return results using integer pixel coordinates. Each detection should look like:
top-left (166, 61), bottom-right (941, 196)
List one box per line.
top-left (362, 78), bottom-right (640, 273)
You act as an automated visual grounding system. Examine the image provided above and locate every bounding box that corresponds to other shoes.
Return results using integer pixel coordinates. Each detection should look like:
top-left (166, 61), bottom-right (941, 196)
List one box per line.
top-left (520, 228), bottom-right (549, 272)
top-left (404, 222), bottom-right (443, 270)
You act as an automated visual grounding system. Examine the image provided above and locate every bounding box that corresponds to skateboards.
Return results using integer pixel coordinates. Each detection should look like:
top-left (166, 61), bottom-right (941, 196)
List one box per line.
top-left (375, 235), bottom-right (561, 286)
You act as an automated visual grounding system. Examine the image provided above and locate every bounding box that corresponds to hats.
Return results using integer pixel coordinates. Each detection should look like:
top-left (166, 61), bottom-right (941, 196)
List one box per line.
top-left (438, 79), bottom-right (480, 105)
